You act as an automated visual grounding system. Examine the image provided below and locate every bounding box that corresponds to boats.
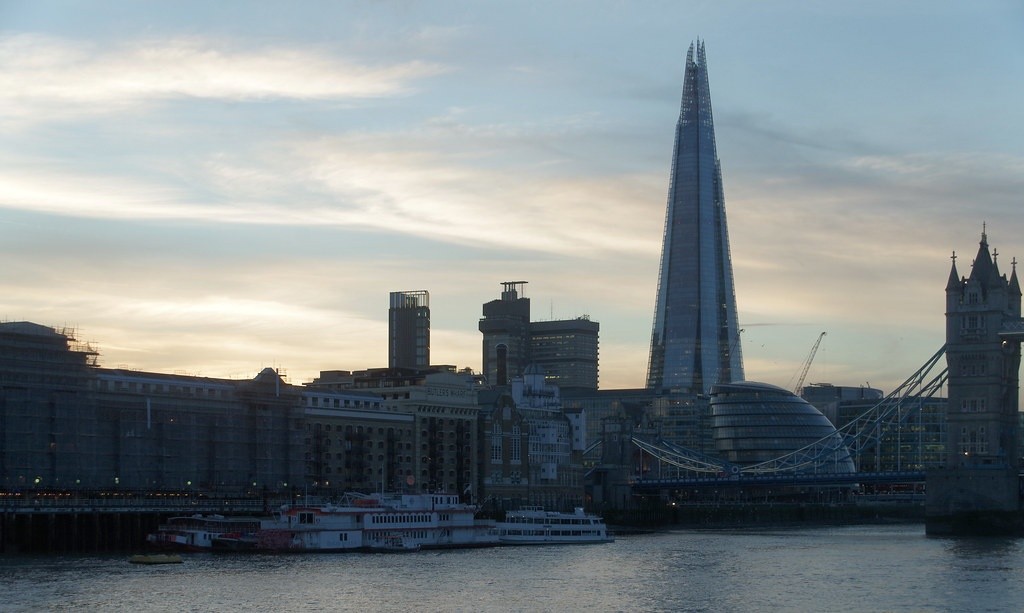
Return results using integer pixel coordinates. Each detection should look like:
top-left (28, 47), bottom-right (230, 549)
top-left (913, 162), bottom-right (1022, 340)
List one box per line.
top-left (502, 505), bottom-right (616, 545)
top-left (144, 480), bottom-right (505, 555)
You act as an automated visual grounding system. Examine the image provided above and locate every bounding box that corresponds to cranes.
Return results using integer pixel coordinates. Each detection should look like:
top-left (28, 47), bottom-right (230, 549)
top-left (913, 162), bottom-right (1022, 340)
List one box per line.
top-left (785, 331), bottom-right (828, 397)
